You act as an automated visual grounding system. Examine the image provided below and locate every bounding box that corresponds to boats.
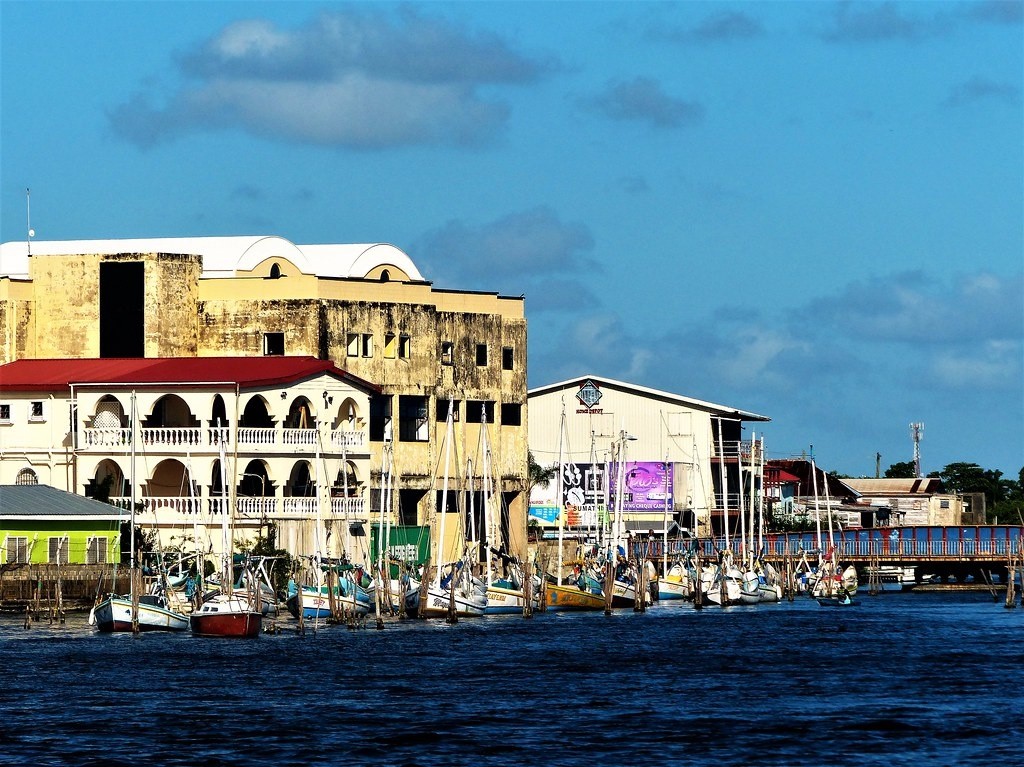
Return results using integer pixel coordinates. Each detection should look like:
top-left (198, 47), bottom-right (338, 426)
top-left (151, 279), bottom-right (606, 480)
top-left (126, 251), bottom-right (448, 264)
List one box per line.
top-left (818, 598), bottom-right (861, 607)
top-left (858, 567), bottom-right (904, 593)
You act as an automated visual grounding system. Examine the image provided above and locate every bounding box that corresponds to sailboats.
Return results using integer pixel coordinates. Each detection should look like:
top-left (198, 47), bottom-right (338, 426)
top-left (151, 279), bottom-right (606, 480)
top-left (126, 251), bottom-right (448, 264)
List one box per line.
top-left (92, 389), bottom-right (859, 632)
